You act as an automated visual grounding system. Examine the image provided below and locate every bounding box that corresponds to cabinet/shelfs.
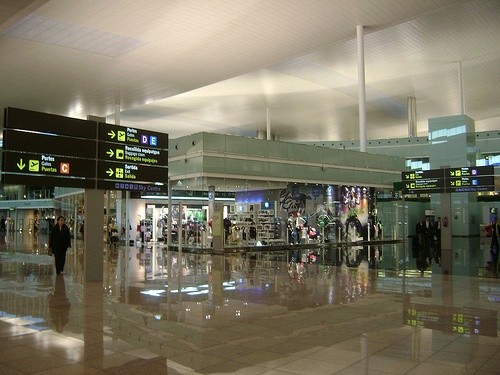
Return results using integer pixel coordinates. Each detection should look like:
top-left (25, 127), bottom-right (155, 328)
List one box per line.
top-left (225, 204), bottom-right (274, 245)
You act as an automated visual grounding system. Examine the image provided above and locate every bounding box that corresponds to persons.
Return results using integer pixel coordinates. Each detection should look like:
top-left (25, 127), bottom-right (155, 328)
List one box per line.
top-left (492, 217), bottom-right (500, 240)
top-left (378, 219), bottom-right (382, 239)
top-left (145, 228), bottom-right (151, 241)
top-left (421, 217), bottom-right (441, 244)
top-left (108, 221), bottom-right (117, 246)
top-left (288, 213), bottom-right (306, 245)
top-left (51, 218), bottom-right (72, 275)
top-left (224, 214), bottom-right (231, 240)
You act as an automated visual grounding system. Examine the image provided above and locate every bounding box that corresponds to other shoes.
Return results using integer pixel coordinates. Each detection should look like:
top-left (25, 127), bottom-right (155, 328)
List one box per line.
top-left (56, 270), bottom-right (61, 275)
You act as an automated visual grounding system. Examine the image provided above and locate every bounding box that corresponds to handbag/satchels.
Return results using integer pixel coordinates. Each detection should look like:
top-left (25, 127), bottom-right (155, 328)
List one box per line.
top-left (46, 248), bottom-right (53, 256)
